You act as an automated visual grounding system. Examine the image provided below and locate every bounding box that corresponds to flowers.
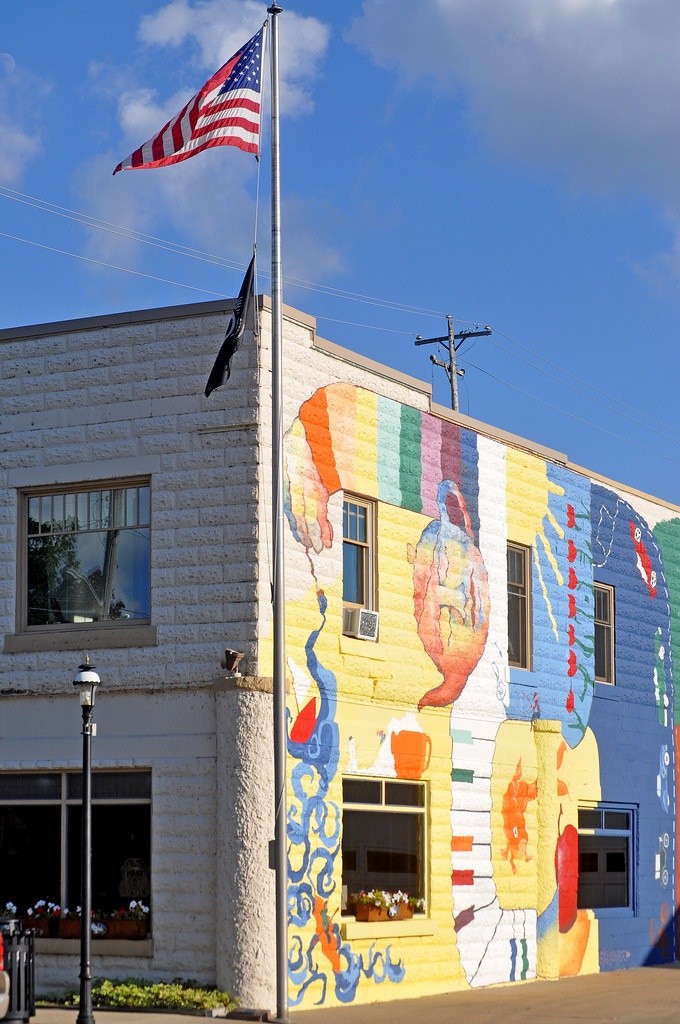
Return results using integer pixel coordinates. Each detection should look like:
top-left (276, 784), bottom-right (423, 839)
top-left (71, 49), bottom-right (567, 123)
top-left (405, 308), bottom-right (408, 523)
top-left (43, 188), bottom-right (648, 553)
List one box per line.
top-left (347, 888), bottom-right (409, 913)
top-left (24, 899), bottom-right (62, 919)
top-left (408, 897), bottom-right (426, 914)
top-left (58, 905), bottom-right (102, 921)
top-left (101, 900), bottom-right (151, 921)
top-left (0, 901), bottom-right (23, 920)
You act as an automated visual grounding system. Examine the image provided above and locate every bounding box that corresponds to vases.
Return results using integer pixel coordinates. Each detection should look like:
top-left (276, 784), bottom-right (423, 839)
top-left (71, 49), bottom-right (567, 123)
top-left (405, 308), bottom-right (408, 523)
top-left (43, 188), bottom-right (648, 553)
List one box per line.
top-left (404, 902), bottom-right (415, 919)
top-left (354, 901), bottom-right (405, 921)
top-left (59, 920), bottom-right (102, 940)
top-left (21, 918), bottom-right (60, 939)
top-left (104, 919), bottom-right (146, 941)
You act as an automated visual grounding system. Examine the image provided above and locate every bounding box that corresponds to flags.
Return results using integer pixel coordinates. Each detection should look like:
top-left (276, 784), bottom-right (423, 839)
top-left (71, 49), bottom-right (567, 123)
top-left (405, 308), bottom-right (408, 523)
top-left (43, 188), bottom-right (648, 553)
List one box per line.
top-left (204, 256), bottom-right (254, 399)
top-left (110, 18), bottom-right (263, 176)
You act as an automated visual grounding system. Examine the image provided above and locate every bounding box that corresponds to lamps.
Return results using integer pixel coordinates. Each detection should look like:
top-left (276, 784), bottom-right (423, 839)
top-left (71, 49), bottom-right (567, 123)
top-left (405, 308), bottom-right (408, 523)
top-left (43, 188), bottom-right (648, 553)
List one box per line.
top-left (225, 648), bottom-right (246, 678)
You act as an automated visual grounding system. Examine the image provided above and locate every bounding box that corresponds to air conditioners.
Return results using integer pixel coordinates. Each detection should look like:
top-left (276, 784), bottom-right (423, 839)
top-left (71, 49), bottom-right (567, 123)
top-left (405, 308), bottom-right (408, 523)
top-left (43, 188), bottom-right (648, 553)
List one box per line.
top-left (343, 607), bottom-right (379, 641)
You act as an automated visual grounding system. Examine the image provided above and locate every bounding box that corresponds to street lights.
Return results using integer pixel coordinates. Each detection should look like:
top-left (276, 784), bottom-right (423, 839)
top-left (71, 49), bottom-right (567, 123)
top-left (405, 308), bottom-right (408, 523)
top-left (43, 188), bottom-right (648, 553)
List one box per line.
top-left (72, 653), bottom-right (102, 1024)
